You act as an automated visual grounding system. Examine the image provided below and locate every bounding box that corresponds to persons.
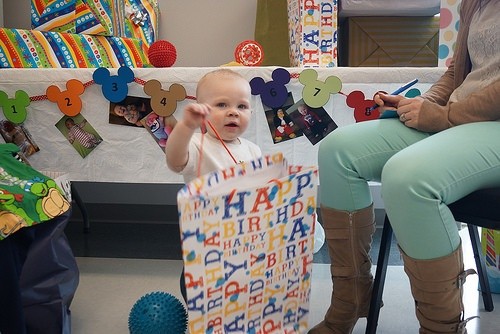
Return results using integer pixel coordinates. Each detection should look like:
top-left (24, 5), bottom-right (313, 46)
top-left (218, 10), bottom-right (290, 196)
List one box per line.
top-left (271, 108), bottom-right (297, 142)
top-left (145, 109), bottom-right (172, 148)
top-left (115, 101), bottom-right (149, 127)
top-left (166, 69), bottom-right (262, 304)
top-left (297, 104), bottom-right (327, 140)
top-left (63, 118), bottom-right (101, 148)
top-left (299, 0), bottom-right (500, 334)
top-left (1, 119), bottom-right (33, 157)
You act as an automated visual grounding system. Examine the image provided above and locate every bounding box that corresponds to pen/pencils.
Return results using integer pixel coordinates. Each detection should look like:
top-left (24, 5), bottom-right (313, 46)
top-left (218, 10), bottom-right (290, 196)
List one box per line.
top-left (370, 79), bottom-right (418, 110)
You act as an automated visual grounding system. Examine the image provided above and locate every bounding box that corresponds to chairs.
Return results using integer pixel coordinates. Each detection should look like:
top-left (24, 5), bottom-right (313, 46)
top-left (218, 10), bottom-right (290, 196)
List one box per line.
top-left (365, 187), bottom-right (500, 334)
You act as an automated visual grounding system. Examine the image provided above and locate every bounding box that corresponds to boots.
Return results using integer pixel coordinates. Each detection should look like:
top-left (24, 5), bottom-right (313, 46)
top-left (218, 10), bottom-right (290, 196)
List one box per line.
top-left (397, 236), bottom-right (480, 334)
top-left (307, 201), bottom-right (384, 334)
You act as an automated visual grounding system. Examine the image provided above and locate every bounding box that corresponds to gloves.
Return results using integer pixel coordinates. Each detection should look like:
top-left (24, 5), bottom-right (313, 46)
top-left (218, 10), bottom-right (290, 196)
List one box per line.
top-left (277, 125), bottom-right (285, 134)
top-left (289, 121), bottom-right (294, 128)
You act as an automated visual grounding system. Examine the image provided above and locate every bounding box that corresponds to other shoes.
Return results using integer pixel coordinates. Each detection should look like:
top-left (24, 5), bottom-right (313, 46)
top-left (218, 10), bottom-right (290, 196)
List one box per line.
top-left (275, 137), bottom-right (282, 143)
top-left (289, 133), bottom-right (296, 138)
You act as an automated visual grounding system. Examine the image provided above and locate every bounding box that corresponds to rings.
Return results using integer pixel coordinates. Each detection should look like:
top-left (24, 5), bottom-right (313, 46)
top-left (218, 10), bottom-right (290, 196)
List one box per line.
top-left (400, 113), bottom-right (407, 122)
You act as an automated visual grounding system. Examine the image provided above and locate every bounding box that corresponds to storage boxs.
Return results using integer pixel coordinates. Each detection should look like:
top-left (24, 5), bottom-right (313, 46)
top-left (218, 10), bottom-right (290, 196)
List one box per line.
top-left (348, 16), bottom-right (440, 66)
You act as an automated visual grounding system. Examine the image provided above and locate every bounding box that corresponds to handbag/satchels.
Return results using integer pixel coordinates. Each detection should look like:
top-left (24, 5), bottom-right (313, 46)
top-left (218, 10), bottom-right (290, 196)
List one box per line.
top-left (177, 115), bottom-right (318, 334)
top-left (0, 185), bottom-right (90, 334)
top-left (478, 227), bottom-right (500, 294)
